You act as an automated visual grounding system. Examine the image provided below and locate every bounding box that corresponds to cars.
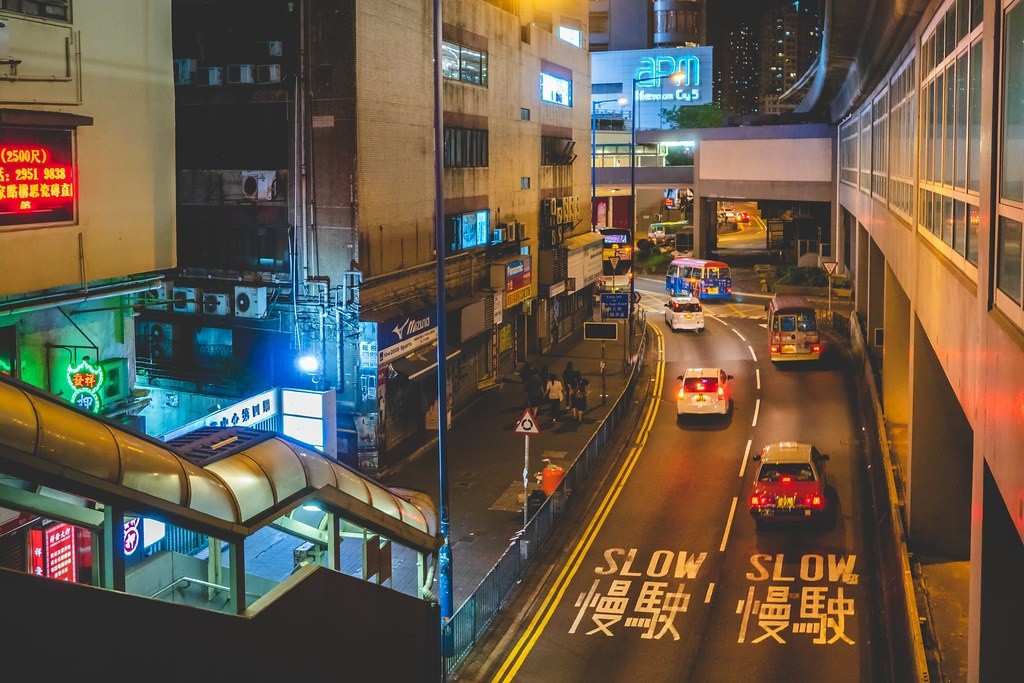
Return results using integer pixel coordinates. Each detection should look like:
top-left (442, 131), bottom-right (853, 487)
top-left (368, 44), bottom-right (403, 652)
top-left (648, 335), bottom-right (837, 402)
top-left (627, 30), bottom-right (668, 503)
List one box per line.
top-left (735, 212), bottom-right (749, 222)
top-left (676, 368), bottom-right (734, 416)
top-left (749, 441), bottom-right (830, 530)
top-left (724, 209), bottom-right (734, 218)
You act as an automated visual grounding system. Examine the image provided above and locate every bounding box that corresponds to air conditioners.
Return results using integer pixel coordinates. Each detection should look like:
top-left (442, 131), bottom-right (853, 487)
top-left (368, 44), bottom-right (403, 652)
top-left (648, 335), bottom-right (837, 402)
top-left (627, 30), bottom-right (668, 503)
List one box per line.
top-left (493, 228), bottom-right (506, 242)
top-left (202, 292), bottom-right (230, 315)
top-left (233, 285), bottom-right (267, 319)
top-left (227, 64), bottom-right (255, 84)
top-left (242, 172), bottom-right (277, 200)
top-left (173, 58), bottom-right (197, 85)
top-left (196, 66), bottom-right (223, 86)
top-left (516, 222), bottom-right (526, 240)
top-left (500, 221), bottom-right (516, 242)
top-left (256, 64), bottom-right (281, 83)
top-left (544, 195), bottom-right (580, 245)
top-left (172, 286), bottom-right (201, 312)
top-left (144, 281), bottom-right (175, 310)
top-left (258, 40), bottom-right (283, 56)
top-left (95, 357), bottom-right (130, 404)
top-left (140, 323), bottom-right (174, 357)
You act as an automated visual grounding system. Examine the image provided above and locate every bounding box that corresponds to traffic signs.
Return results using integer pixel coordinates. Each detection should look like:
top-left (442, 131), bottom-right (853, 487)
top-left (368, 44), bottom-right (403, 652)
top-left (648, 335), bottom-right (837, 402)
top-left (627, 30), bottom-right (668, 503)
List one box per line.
top-left (624, 292), bottom-right (641, 303)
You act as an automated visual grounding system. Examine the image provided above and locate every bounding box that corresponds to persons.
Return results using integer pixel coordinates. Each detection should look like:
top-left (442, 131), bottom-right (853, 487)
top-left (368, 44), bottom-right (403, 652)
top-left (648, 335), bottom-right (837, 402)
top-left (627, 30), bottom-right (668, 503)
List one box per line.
top-left (519, 362), bottom-right (591, 422)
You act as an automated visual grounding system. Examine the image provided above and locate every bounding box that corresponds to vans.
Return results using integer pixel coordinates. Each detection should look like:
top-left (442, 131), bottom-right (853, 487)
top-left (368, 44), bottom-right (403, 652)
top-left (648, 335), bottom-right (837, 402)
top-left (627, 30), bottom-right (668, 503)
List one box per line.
top-left (664, 297), bottom-right (704, 333)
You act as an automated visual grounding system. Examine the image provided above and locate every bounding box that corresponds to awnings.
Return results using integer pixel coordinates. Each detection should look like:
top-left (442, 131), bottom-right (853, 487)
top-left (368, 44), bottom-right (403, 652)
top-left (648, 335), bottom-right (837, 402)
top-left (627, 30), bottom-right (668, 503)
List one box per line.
top-left (392, 341), bottom-right (461, 380)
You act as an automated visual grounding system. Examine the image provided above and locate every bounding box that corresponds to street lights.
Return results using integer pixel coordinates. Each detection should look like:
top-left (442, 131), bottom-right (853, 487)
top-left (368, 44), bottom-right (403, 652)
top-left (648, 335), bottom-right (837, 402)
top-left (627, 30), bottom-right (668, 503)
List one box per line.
top-left (593, 97), bottom-right (627, 232)
top-left (612, 245), bottom-right (629, 293)
top-left (631, 74), bottom-right (688, 363)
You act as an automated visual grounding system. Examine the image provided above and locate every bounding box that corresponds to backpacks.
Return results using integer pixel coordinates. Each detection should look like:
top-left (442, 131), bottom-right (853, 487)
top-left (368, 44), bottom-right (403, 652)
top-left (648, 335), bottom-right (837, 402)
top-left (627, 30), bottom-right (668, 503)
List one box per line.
top-left (574, 377), bottom-right (585, 398)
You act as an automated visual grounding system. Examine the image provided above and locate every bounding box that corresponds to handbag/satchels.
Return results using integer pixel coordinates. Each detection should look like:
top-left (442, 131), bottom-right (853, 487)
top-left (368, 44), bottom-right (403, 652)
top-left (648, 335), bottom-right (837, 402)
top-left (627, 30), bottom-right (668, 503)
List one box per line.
top-left (560, 392), bottom-right (563, 401)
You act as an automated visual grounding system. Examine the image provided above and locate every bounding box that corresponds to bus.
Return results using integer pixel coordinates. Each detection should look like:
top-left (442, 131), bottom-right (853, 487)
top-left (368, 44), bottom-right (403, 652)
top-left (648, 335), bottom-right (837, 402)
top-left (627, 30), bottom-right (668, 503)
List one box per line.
top-left (665, 258), bottom-right (731, 304)
top-left (596, 227), bottom-right (631, 262)
top-left (764, 296), bottom-right (821, 365)
top-left (648, 222), bottom-right (687, 246)
